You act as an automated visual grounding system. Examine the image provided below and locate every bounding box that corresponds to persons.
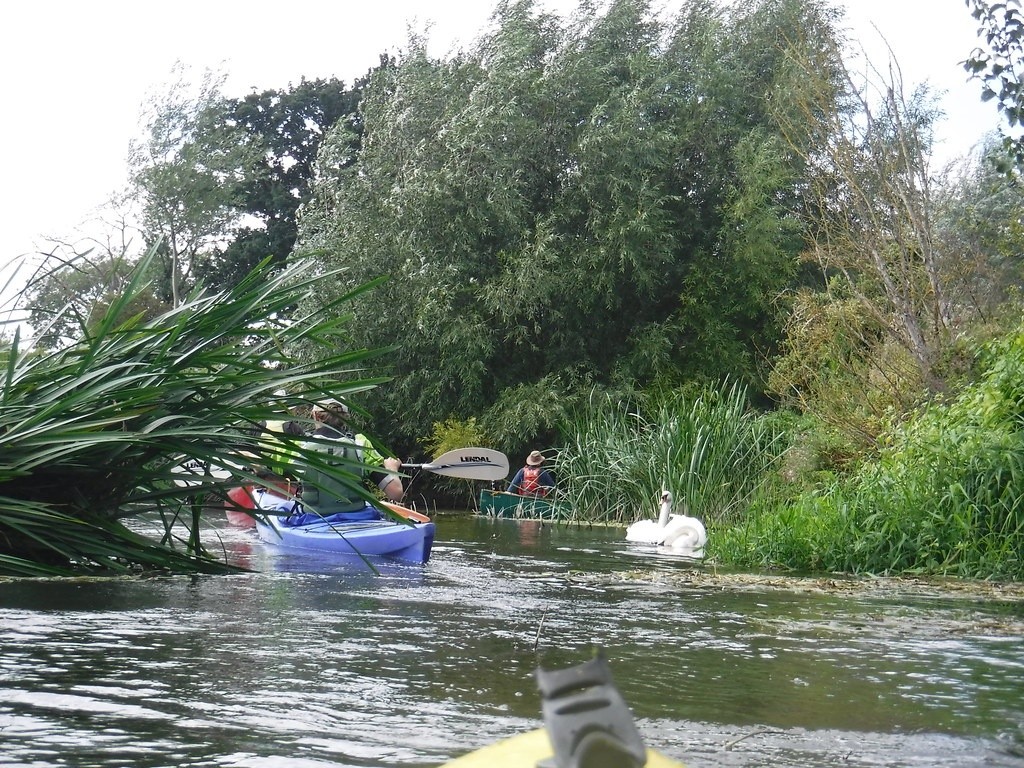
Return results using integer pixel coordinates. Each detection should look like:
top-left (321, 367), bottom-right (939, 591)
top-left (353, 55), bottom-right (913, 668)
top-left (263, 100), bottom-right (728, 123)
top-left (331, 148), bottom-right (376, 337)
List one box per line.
top-left (507, 451), bottom-right (556, 498)
top-left (233, 390), bottom-right (403, 514)
top-left (257, 390), bottom-right (311, 483)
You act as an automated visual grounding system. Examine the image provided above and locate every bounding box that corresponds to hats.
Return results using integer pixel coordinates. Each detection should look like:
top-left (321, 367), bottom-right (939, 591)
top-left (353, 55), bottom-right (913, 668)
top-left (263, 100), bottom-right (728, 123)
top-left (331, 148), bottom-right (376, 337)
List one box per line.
top-left (526, 450), bottom-right (545, 466)
top-left (312, 391), bottom-right (349, 413)
top-left (264, 390), bottom-right (304, 413)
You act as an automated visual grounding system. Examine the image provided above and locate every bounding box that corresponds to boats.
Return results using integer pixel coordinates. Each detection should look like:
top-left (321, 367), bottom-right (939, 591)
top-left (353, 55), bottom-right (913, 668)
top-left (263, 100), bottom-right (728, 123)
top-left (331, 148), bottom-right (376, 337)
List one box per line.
top-left (479, 488), bottom-right (573, 519)
top-left (251, 487), bottom-right (435, 563)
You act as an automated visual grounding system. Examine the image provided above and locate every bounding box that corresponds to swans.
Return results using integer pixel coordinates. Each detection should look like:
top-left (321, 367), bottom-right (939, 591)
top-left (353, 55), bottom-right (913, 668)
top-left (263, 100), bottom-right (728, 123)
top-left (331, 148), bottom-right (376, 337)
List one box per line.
top-left (625, 490), bottom-right (708, 550)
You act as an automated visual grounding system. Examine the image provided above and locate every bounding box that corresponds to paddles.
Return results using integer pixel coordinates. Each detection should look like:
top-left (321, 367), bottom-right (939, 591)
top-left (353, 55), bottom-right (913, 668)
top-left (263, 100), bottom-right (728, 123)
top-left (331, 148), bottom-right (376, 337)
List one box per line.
top-left (171, 444), bottom-right (510, 483)
